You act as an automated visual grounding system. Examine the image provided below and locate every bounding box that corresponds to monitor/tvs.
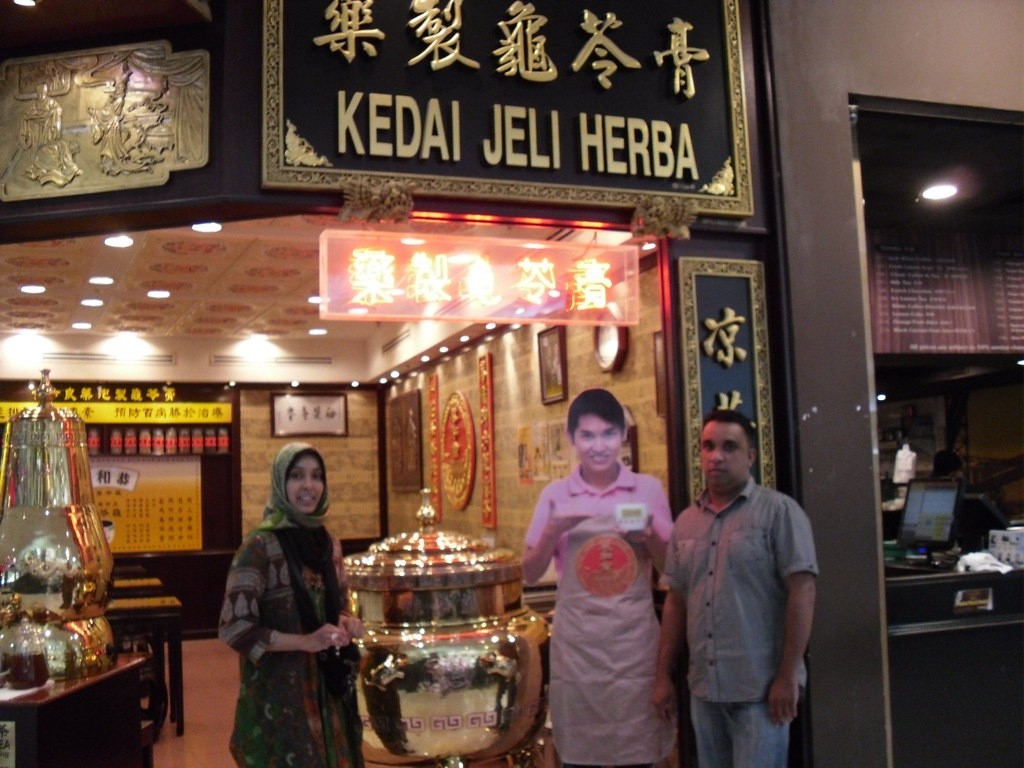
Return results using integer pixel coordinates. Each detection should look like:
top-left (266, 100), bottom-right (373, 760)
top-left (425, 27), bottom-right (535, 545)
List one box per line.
top-left (899, 478), bottom-right (963, 547)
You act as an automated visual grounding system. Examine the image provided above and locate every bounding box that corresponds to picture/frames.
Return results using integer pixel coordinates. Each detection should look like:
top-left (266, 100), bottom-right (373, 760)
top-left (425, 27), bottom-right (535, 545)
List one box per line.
top-left (271, 392), bottom-right (349, 436)
top-left (537, 325), bottom-right (567, 404)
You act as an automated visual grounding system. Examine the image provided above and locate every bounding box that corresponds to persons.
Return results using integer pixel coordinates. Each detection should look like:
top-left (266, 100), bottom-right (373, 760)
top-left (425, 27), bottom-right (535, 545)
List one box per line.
top-left (654, 409), bottom-right (822, 768)
top-left (516, 387), bottom-right (684, 767)
top-left (926, 448), bottom-right (967, 479)
top-left (217, 443), bottom-right (377, 767)
